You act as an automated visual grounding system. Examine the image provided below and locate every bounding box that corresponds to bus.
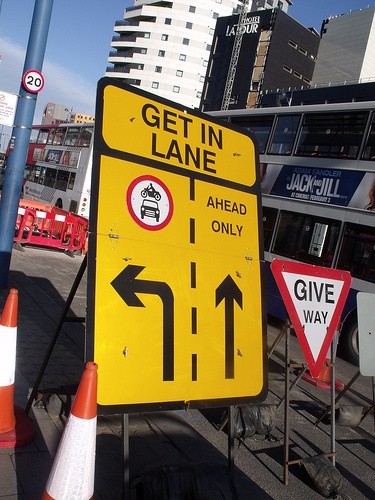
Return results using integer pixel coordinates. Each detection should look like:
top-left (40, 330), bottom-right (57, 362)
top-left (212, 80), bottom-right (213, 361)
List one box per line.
top-left (1, 100), bottom-right (375, 366)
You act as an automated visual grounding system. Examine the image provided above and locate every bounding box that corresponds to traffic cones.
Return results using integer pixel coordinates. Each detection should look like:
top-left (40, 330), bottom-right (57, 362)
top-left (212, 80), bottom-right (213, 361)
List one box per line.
top-left (0, 288), bottom-right (34, 448)
top-left (41, 362), bottom-right (98, 500)
